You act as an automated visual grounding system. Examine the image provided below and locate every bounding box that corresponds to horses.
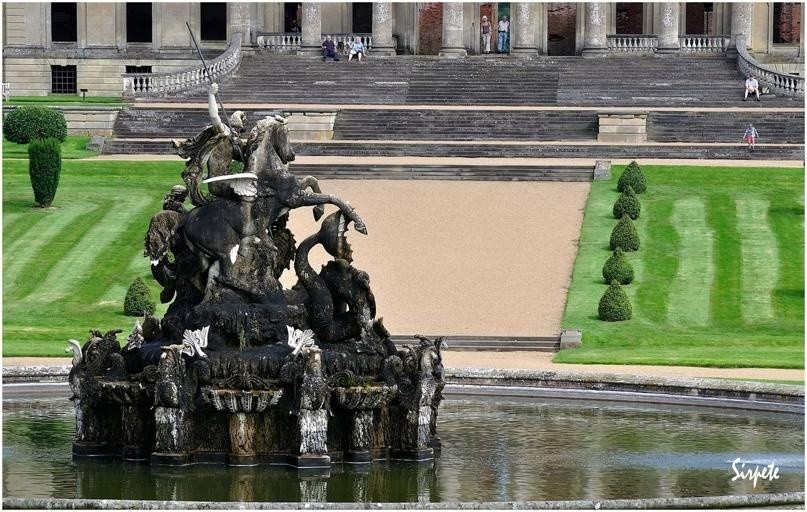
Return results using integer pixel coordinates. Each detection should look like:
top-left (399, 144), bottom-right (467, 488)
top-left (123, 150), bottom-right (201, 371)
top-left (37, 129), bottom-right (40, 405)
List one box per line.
top-left (184, 114), bottom-right (367, 296)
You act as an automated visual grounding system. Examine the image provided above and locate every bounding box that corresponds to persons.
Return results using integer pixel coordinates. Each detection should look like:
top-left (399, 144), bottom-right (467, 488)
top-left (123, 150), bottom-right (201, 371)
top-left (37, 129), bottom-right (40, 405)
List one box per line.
top-left (742, 124), bottom-right (759, 149)
top-left (479, 15), bottom-right (492, 54)
top-left (742, 73), bottom-right (761, 101)
top-left (162, 183), bottom-right (189, 213)
top-left (496, 15), bottom-right (509, 55)
top-left (289, 19), bottom-right (300, 42)
top-left (172, 82), bottom-right (278, 252)
top-left (321, 35), bottom-right (339, 61)
top-left (346, 37), bottom-right (366, 62)
